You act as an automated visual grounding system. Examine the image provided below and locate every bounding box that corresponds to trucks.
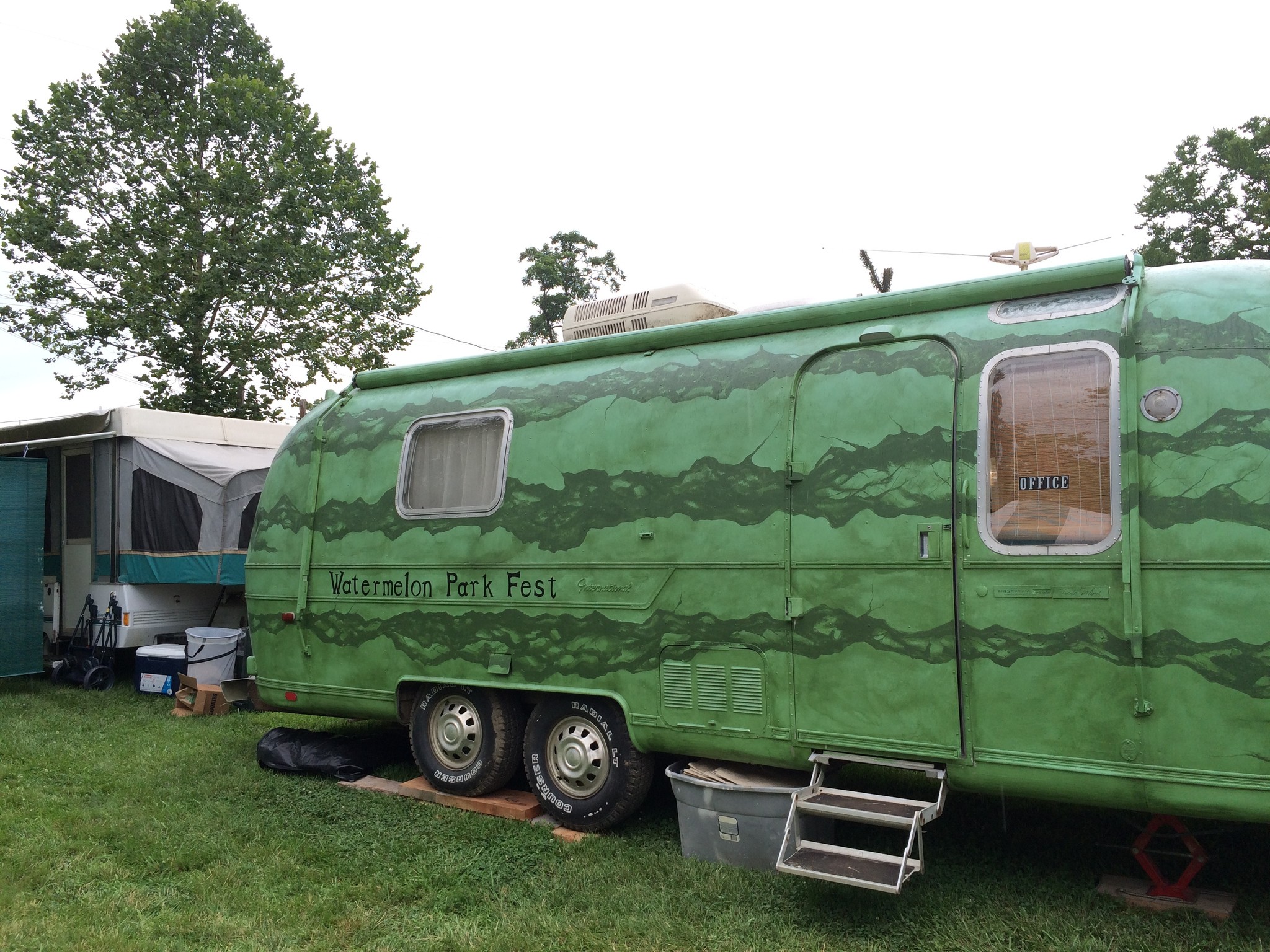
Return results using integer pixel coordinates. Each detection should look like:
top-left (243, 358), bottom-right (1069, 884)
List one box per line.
top-left (0, 408), bottom-right (298, 693)
top-left (242, 255), bottom-right (1268, 893)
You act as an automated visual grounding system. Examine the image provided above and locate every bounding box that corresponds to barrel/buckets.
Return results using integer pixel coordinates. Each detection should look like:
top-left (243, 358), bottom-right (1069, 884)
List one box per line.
top-left (186, 627), bottom-right (241, 687)
